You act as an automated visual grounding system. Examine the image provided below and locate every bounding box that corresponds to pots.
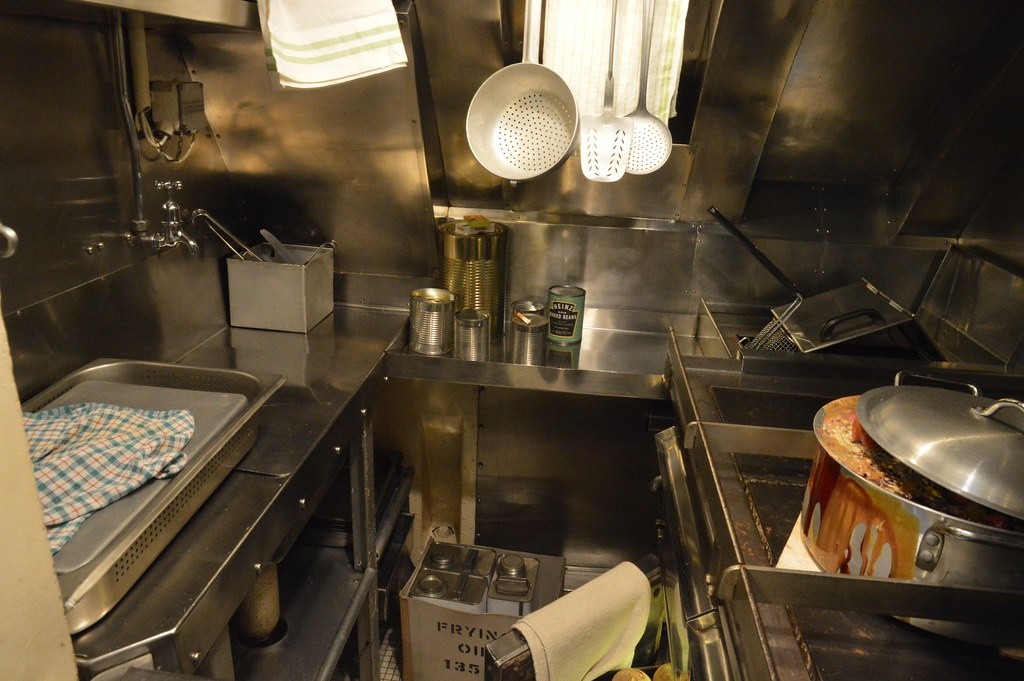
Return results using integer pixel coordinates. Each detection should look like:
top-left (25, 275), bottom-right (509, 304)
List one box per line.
top-left (802, 370), bottom-right (1024, 647)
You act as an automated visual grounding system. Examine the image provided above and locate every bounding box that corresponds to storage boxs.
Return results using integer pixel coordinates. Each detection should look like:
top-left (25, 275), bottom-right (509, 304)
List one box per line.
top-left (399, 537), bottom-right (567, 681)
top-left (227, 243), bottom-right (334, 333)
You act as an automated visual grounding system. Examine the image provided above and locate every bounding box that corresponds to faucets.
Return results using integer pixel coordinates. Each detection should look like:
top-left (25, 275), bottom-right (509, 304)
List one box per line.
top-left (152, 178), bottom-right (201, 256)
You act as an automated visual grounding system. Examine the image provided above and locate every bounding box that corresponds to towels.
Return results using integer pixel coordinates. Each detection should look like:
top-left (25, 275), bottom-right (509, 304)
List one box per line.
top-left (258, 0), bottom-right (408, 94)
